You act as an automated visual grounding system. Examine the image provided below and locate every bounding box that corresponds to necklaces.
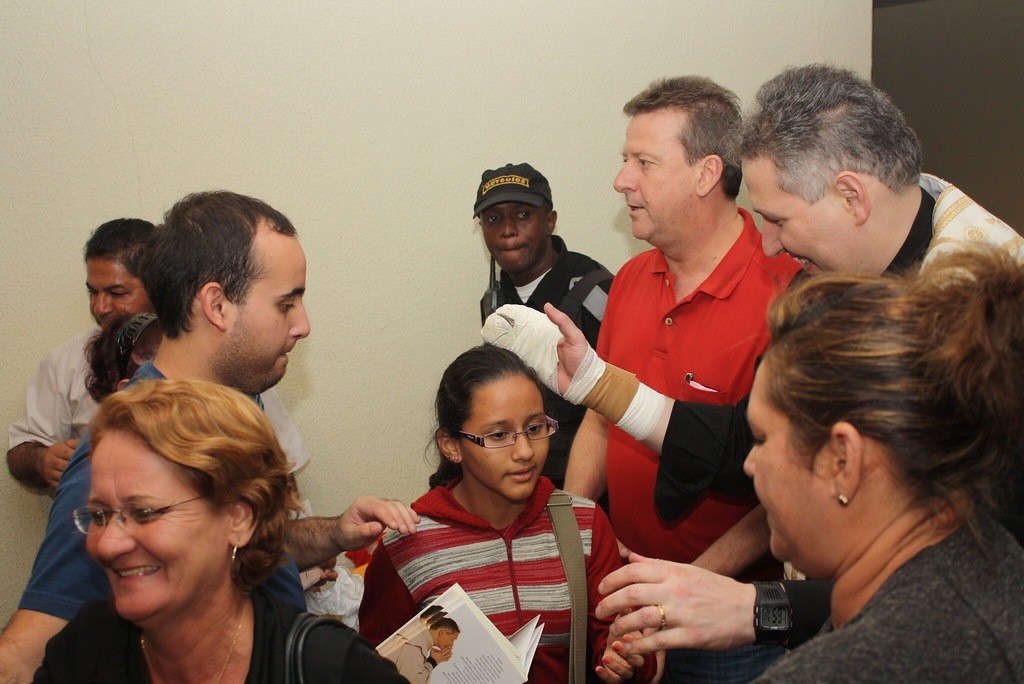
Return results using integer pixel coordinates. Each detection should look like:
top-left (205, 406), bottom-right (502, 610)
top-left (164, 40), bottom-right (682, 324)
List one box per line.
top-left (141, 600), bottom-right (248, 684)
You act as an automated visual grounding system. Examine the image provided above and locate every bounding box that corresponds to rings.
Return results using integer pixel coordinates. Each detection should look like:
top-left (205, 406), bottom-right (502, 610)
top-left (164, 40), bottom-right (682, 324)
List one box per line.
top-left (655, 604), bottom-right (668, 628)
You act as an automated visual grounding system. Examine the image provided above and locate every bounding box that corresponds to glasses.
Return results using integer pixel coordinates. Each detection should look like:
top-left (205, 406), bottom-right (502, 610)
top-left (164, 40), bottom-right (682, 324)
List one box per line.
top-left (70, 494), bottom-right (208, 536)
top-left (458, 416), bottom-right (559, 449)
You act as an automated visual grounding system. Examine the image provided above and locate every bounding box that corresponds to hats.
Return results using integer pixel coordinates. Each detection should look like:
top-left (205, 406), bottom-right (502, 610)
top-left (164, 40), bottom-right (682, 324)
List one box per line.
top-left (472, 163), bottom-right (552, 220)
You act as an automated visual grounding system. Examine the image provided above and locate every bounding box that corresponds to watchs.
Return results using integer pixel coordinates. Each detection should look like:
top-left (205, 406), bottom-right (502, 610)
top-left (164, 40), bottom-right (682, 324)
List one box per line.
top-left (749, 578), bottom-right (791, 647)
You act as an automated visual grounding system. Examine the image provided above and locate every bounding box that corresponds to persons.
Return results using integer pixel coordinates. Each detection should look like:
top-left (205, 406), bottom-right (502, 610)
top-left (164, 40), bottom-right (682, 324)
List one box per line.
top-left (32, 380), bottom-right (414, 684)
top-left (1, 190), bottom-right (421, 684)
top-left (594, 62), bottom-right (1024, 652)
top-left (83, 309), bottom-right (162, 403)
top-left (358, 343), bottom-right (659, 684)
top-left (377, 605), bottom-right (443, 653)
top-left (380, 612), bottom-right (448, 657)
top-left (746, 241), bottom-right (1024, 684)
top-left (6, 219), bottom-right (308, 512)
top-left (472, 161), bottom-right (617, 489)
top-left (385, 618), bottom-right (460, 684)
top-left (561, 73), bottom-right (811, 684)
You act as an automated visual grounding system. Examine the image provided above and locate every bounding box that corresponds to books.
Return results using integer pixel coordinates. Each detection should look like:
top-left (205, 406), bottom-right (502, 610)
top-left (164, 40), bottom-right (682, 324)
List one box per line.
top-left (376, 581), bottom-right (546, 684)
